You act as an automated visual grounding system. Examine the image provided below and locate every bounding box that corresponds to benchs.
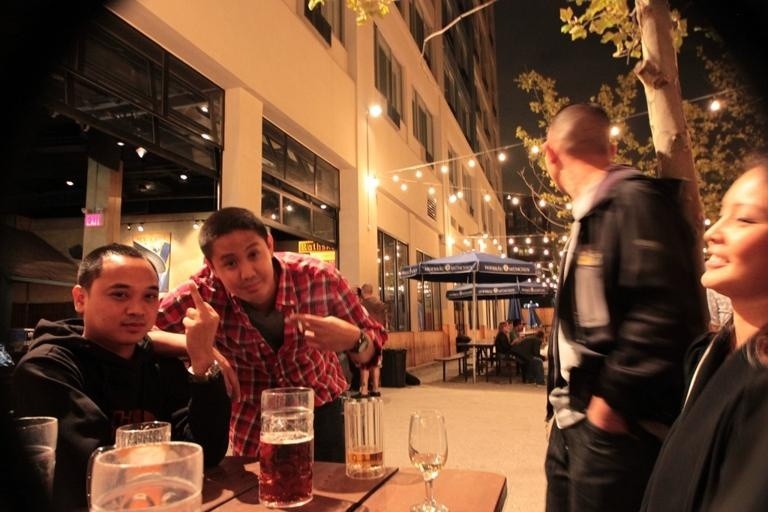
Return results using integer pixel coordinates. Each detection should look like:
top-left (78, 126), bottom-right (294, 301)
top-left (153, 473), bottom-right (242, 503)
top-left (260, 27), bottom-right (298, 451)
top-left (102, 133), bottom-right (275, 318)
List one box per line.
top-left (434, 351), bottom-right (472, 381)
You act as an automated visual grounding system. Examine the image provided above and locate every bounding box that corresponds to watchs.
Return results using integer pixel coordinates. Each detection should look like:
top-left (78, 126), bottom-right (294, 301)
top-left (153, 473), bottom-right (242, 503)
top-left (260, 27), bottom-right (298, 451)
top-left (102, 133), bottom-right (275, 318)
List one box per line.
top-left (351, 329), bottom-right (370, 354)
top-left (186, 361), bottom-right (223, 381)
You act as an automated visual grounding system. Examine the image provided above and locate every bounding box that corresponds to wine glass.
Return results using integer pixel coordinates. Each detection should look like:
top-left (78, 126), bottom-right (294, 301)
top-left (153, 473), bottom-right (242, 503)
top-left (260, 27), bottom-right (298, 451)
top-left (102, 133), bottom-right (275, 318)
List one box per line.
top-left (408, 411), bottom-right (450, 512)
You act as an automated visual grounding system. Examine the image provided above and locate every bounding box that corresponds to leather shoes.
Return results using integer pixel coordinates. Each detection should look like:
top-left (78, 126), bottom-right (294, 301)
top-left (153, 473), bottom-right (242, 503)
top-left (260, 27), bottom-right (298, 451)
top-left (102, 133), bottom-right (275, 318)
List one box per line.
top-left (351, 392), bottom-right (368, 399)
top-left (371, 392), bottom-right (380, 397)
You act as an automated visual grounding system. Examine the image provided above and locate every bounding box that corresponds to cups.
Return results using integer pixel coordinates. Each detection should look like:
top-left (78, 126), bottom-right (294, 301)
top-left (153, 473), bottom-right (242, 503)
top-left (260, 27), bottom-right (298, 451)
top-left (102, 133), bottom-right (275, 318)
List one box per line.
top-left (260, 385), bottom-right (314, 510)
top-left (345, 397), bottom-right (389, 480)
top-left (115, 420), bottom-right (171, 449)
top-left (19, 416), bottom-right (58, 500)
top-left (90, 442), bottom-right (209, 512)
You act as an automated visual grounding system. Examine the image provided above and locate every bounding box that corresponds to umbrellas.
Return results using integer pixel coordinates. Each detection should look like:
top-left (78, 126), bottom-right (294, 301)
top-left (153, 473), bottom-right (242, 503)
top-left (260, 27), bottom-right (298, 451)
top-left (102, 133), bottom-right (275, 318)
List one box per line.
top-left (447, 281), bottom-right (553, 376)
top-left (523, 300), bottom-right (543, 328)
top-left (400, 250), bottom-right (536, 384)
top-left (507, 297), bottom-right (522, 328)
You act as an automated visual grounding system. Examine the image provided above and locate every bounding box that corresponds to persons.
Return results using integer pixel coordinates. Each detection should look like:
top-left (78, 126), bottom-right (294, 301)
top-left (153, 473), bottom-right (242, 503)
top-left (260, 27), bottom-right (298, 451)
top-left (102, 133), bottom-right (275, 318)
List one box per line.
top-left (512, 331), bottom-right (547, 385)
top-left (151, 207), bottom-right (388, 464)
top-left (543, 102), bottom-right (709, 512)
top-left (11, 242), bottom-right (232, 477)
top-left (351, 283), bottom-right (387, 398)
top-left (495, 322), bottom-right (513, 353)
top-left (349, 287), bottom-right (371, 391)
top-left (639, 166), bottom-right (768, 512)
top-left (512, 319), bottom-right (523, 339)
top-left (507, 318), bottom-right (520, 346)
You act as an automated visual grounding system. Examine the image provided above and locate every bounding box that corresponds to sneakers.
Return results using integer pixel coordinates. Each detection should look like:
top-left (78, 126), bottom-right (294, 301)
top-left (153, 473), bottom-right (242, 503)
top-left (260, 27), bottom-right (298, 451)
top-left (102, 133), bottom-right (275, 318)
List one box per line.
top-left (538, 382), bottom-right (548, 387)
top-left (529, 378), bottom-right (535, 382)
